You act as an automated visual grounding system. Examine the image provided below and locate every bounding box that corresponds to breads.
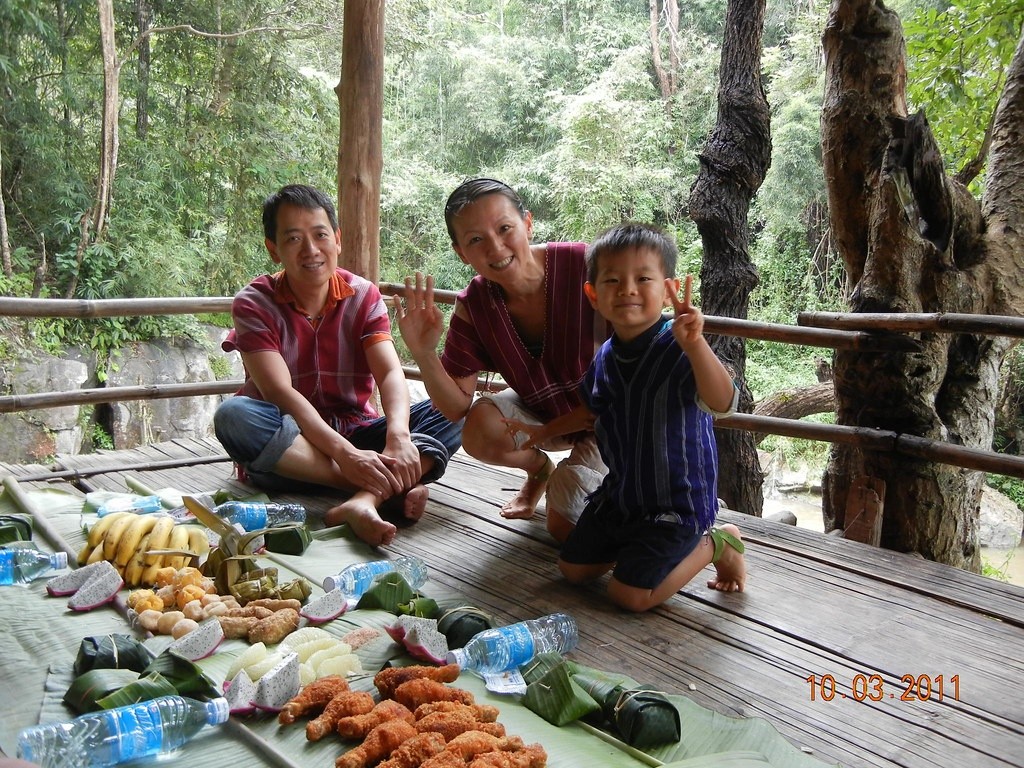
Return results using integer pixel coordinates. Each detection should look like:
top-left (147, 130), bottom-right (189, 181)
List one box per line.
top-left (211, 599), bottom-right (301, 643)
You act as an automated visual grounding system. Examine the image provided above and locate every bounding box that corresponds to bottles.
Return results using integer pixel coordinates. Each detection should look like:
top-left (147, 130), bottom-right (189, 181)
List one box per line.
top-left (194, 502), bottom-right (306, 532)
top-left (16, 695), bottom-right (230, 768)
top-left (323, 557), bottom-right (428, 599)
top-left (0, 547), bottom-right (68, 586)
top-left (448, 613), bottom-right (579, 672)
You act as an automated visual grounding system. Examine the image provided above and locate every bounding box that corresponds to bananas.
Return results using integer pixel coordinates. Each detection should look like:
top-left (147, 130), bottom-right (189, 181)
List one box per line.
top-left (81, 514), bottom-right (208, 586)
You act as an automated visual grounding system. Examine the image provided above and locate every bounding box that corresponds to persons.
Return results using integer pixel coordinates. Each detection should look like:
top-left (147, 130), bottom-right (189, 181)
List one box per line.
top-left (392, 179), bottom-right (616, 540)
top-left (213, 185), bottom-right (466, 547)
top-left (500, 221), bottom-right (746, 611)
